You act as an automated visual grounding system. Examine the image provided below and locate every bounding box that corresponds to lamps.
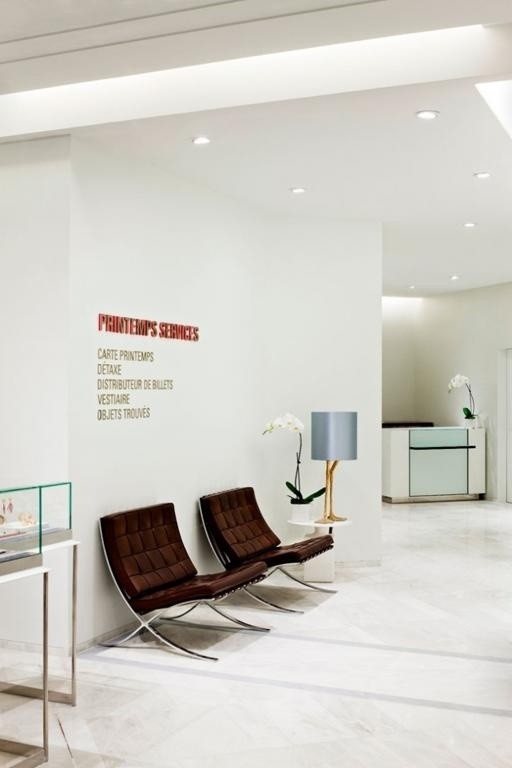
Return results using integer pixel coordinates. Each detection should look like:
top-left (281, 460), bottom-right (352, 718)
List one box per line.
top-left (310, 411), bottom-right (357, 523)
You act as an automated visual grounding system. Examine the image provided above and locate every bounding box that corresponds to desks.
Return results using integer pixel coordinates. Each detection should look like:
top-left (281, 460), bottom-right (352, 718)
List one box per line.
top-left (289, 519), bottom-right (348, 584)
top-left (0, 539), bottom-right (84, 768)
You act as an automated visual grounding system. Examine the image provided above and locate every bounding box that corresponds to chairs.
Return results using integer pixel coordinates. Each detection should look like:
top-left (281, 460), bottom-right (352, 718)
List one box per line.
top-left (198, 487), bottom-right (334, 614)
top-left (99, 501), bottom-right (269, 659)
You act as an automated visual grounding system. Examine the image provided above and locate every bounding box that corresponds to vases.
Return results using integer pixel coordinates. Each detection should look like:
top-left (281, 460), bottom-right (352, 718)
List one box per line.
top-left (462, 419), bottom-right (477, 429)
top-left (290, 504), bottom-right (310, 522)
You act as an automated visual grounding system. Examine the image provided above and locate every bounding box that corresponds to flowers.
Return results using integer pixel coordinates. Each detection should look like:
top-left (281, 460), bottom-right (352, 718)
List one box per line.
top-left (448, 374), bottom-right (479, 419)
top-left (262, 411), bottom-right (327, 505)
